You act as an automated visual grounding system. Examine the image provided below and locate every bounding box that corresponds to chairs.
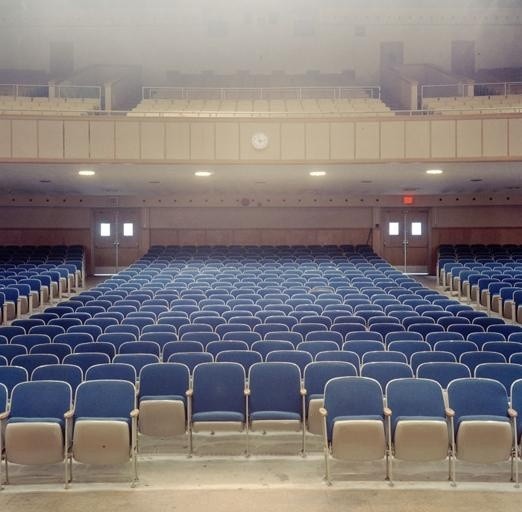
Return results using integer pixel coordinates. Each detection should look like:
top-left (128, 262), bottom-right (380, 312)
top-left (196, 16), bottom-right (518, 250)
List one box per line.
top-left (1, 242), bottom-right (522, 492)
top-left (2, 68), bottom-right (521, 117)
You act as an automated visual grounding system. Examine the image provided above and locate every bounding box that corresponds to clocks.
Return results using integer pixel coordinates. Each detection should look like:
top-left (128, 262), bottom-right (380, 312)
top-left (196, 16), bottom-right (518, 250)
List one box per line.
top-left (251, 132), bottom-right (269, 152)
